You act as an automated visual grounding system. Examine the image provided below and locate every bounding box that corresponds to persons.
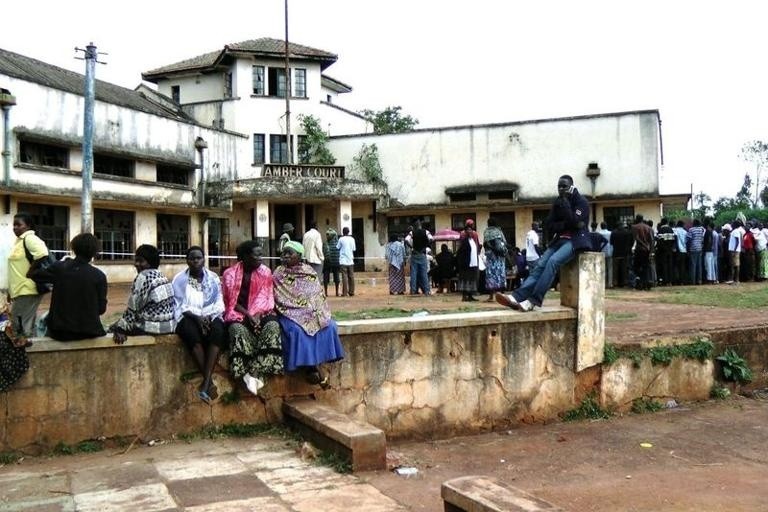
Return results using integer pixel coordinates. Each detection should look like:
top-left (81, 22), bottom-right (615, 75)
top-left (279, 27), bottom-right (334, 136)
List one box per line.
top-left (224, 240), bottom-right (285, 395)
top-left (107, 244), bottom-right (175, 344)
top-left (272, 240), bottom-right (336, 389)
top-left (427, 217), bottom-right (511, 293)
top-left (385, 221), bottom-right (435, 294)
top-left (168, 246), bottom-right (227, 403)
top-left (279, 221), bottom-right (356, 296)
top-left (505, 221), bottom-right (541, 288)
top-left (41, 234), bottom-right (109, 341)
top-left (7, 212), bottom-right (55, 337)
top-left (590, 214), bottom-right (767, 290)
top-left (496, 175), bottom-right (593, 311)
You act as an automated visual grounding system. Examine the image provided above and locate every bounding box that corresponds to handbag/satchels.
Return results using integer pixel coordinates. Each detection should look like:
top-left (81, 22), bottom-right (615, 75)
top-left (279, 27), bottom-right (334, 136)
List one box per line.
top-left (34, 253), bottom-right (57, 294)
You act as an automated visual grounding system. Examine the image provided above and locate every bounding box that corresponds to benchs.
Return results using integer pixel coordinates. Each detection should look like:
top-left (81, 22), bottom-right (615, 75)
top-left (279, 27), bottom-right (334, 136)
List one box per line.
top-left (439, 275), bottom-right (517, 293)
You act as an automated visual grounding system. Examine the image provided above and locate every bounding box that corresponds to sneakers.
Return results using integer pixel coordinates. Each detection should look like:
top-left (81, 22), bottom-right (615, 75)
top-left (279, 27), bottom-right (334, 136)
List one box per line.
top-left (496, 292), bottom-right (534, 312)
top-left (243, 372), bottom-right (264, 396)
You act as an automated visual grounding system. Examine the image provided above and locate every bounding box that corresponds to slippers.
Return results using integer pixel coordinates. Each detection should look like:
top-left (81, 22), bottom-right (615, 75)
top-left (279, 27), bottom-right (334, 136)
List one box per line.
top-left (200, 385), bottom-right (218, 403)
